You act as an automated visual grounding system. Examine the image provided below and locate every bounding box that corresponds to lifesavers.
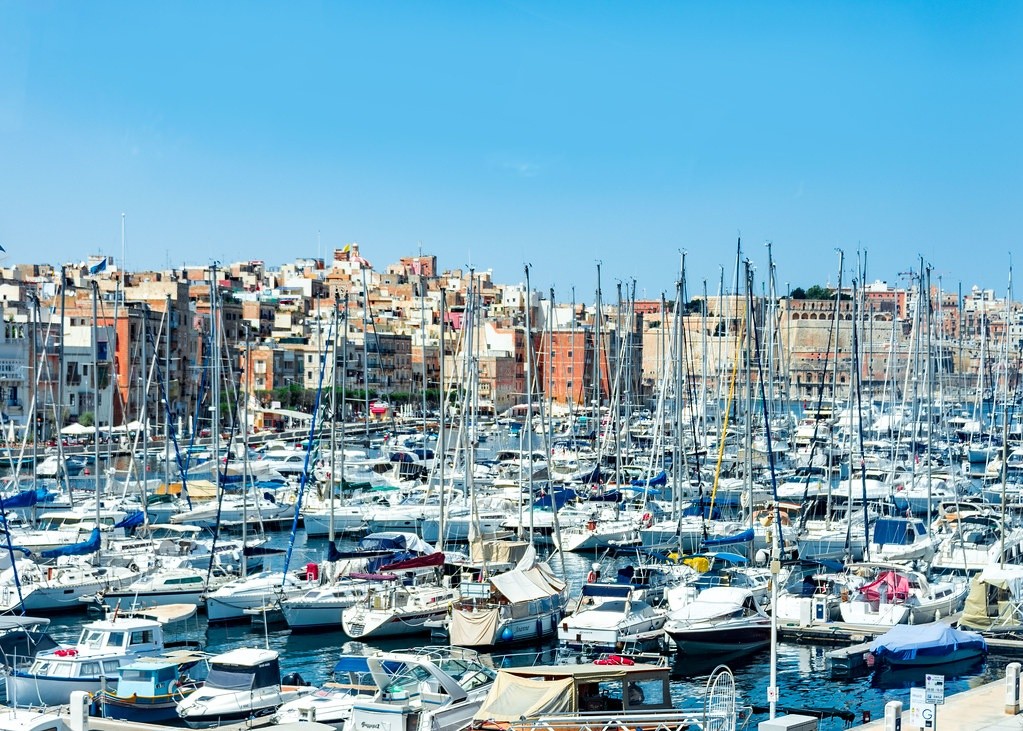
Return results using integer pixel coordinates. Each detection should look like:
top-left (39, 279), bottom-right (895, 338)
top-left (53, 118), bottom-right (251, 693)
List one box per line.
top-left (168, 679), bottom-right (183, 694)
top-left (54, 649), bottom-right (79, 656)
top-left (587, 570), bottom-right (597, 584)
top-left (594, 655), bottom-right (634, 666)
top-left (643, 513), bottom-right (652, 528)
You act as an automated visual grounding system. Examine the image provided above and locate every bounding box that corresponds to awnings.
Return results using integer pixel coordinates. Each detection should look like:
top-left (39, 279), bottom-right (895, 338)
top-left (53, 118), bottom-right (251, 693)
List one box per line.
top-left (0, 401), bottom-right (1023, 731)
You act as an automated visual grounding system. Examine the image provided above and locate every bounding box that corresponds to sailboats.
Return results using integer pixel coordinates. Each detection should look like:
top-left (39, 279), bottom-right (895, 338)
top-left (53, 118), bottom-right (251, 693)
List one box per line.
top-left (0, 235), bottom-right (1020, 731)
